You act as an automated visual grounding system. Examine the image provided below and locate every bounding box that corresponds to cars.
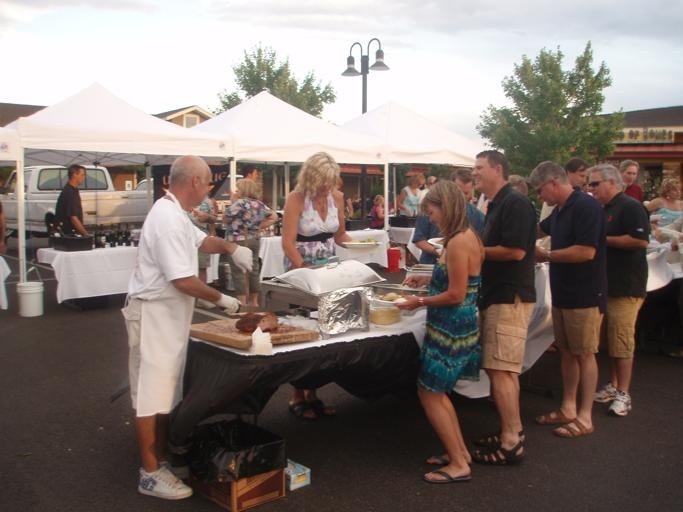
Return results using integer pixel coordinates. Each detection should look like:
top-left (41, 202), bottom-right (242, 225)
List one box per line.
top-left (129, 175), bottom-right (246, 202)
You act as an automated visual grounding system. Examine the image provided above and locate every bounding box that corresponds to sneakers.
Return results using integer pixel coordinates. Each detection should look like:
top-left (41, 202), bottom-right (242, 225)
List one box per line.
top-left (138, 461), bottom-right (194, 500)
top-left (608, 389), bottom-right (632, 416)
top-left (593, 382), bottom-right (617, 403)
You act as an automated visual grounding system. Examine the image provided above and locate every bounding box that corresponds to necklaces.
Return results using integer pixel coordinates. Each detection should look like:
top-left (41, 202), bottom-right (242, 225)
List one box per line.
top-left (314, 197), bottom-right (326, 214)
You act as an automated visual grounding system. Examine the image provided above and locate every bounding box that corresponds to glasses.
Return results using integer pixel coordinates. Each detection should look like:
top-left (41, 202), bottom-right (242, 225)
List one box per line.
top-left (535, 177), bottom-right (559, 194)
top-left (588, 179), bottom-right (607, 188)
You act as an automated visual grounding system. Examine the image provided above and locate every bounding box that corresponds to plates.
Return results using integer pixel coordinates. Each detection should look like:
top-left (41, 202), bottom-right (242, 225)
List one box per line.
top-left (372, 297), bottom-right (398, 304)
top-left (427, 237), bottom-right (445, 249)
top-left (342, 242), bottom-right (382, 247)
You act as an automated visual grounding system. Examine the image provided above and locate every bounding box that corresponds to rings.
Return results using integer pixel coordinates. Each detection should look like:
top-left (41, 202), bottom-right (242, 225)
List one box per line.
top-left (407, 306), bottom-right (409, 310)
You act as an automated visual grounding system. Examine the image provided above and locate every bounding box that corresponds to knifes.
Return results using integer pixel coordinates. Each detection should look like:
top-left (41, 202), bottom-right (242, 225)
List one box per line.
top-left (221, 305), bottom-right (284, 314)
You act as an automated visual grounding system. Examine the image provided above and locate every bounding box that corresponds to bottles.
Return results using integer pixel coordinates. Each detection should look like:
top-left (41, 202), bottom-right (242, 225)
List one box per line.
top-left (47, 222), bottom-right (77, 237)
top-left (94, 223), bottom-right (130, 248)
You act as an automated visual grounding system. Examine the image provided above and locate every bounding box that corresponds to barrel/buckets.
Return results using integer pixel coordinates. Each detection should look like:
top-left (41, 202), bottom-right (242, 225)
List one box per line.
top-left (285, 458), bottom-right (311, 491)
top-left (16, 265), bottom-right (45, 317)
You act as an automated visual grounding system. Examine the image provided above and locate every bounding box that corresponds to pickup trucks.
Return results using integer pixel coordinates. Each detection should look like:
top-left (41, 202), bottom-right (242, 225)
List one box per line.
top-left (1, 165), bottom-right (154, 237)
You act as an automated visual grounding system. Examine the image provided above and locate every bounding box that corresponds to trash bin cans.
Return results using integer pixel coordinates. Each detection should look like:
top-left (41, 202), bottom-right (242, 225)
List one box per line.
top-left (383, 249), bottom-right (399, 273)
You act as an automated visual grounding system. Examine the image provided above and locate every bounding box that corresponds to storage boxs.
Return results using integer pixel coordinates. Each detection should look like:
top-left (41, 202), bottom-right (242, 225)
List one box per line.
top-left (187, 420), bottom-right (287, 512)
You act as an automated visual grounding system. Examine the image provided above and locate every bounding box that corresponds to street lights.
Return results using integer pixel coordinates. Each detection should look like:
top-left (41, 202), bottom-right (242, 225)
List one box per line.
top-left (340, 36), bottom-right (388, 228)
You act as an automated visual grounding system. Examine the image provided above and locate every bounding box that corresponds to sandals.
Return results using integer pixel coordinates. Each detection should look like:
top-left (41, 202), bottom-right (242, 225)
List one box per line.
top-left (472, 440), bottom-right (526, 465)
top-left (473, 429), bottom-right (526, 446)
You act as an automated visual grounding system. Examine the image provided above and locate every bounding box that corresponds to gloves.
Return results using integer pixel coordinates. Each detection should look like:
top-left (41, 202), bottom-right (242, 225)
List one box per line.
top-left (213, 294), bottom-right (243, 314)
top-left (231, 244), bottom-right (254, 275)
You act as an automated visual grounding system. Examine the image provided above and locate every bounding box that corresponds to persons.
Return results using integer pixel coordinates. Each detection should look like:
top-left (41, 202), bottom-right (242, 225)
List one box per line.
top-left (0, 200), bottom-right (6, 255)
top-left (592, 162), bottom-right (652, 419)
top-left (280, 150), bottom-right (356, 420)
top-left (192, 203), bottom-right (214, 281)
top-left (472, 150), bottom-right (537, 466)
top-left (529, 158), bottom-right (605, 439)
top-left (220, 177), bottom-right (280, 306)
top-left (413, 179), bottom-right (487, 484)
top-left (55, 163), bottom-right (87, 238)
top-left (118, 153), bottom-right (254, 502)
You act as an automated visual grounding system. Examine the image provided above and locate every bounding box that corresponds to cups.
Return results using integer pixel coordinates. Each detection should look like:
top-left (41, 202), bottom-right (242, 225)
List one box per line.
top-left (255, 222), bottom-right (282, 240)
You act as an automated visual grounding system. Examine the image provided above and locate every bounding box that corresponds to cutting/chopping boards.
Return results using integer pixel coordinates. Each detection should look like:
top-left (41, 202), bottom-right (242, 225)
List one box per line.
top-left (188, 318), bottom-right (320, 349)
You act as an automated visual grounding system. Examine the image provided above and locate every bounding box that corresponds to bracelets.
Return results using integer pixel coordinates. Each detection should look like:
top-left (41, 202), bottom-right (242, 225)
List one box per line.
top-left (417, 297), bottom-right (423, 306)
top-left (540, 249), bottom-right (551, 262)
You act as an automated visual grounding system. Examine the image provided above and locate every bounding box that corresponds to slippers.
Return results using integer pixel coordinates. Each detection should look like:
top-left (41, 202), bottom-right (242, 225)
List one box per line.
top-left (552, 418), bottom-right (594, 439)
top-left (426, 455), bottom-right (474, 465)
top-left (423, 469), bottom-right (472, 483)
top-left (535, 408), bottom-right (577, 425)
top-left (289, 399), bottom-right (336, 420)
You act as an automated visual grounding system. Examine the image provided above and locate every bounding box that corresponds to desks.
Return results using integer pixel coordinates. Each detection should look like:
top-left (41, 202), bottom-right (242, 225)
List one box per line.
top-left (36, 247), bottom-right (220, 311)
top-left (390, 226), bottom-right (424, 262)
top-left (258, 229), bottom-right (388, 281)
top-left (188, 249), bottom-right (683, 426)
